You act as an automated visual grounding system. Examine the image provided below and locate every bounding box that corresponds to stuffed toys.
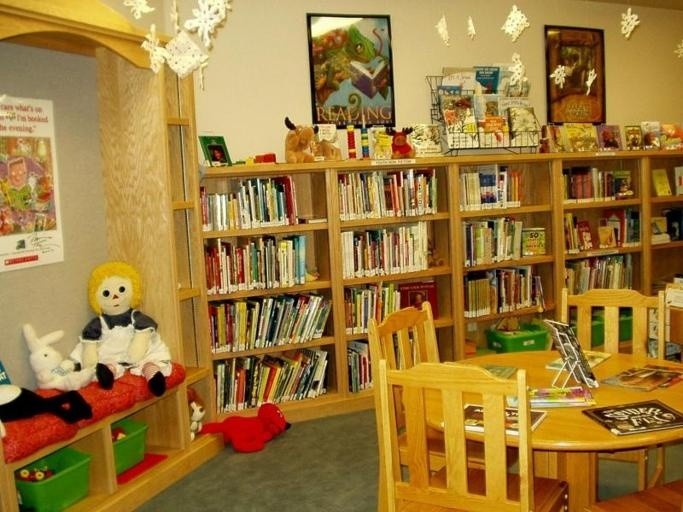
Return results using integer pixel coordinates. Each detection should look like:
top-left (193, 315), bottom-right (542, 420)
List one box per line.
top-left (283, 117), bottom-right (320, 163)
top-left (386, 127), bottom-right (413, 158)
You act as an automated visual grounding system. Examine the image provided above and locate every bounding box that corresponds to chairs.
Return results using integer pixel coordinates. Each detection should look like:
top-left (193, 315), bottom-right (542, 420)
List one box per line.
top-left (366, 288), bottom-right (683, 512)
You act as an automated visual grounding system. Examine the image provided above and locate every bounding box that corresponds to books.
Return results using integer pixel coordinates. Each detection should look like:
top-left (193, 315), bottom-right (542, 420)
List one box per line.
top-left (542, 320), bottom-right (599, 388)
top-left (338, 168), bottom-right (437, 392)
top-left (442, 404), bottom-right (547, 436)
top-left (308, 123), bottom-right (444, 162)
top-left (437, 64), bottom-right (539, 148)
top-left (563, 165), bottom-right (641, 295)
top-left (545, 350), bottom-right (611, 372)
top-left (541, 121), bottom-right (683, 154)
top-left (583, 400), bottom-right (683, 436)
top-left (459, 164), bottom-right (546, 331)
top-left (196, 170), bottom-right (333, 411)
top-left (650, 167), bottom-right (682, 245)
top-left (599, 363), bottom-right (682, 392)
top-left (508, 385), bottom-right (597, 407)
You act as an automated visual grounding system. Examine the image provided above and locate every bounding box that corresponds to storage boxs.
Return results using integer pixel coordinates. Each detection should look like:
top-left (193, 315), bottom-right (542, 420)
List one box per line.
top-left (15, 448), bottom-right (93, 511)
top-left (111, 420), bottom-right (148, 476)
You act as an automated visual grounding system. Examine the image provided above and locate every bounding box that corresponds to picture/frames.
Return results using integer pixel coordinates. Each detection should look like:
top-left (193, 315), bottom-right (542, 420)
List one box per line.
top-left (544, 25), bottom-right (606, 125)
top-left (306, 13), bottom-right (395, 128)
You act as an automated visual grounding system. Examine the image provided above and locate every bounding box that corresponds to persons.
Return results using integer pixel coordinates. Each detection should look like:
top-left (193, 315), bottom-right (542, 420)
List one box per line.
top-left (7, 158), bottom-right (32, 232)
top-left (602, 125), bottom-right (619, 148)
top-left (214, 149), bottom-right (224, 162)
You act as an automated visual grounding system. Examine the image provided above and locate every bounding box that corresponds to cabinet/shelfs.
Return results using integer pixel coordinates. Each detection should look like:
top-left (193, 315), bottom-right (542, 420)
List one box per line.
top-left (200, 148), bottom-right (683, 426)
top-left (0, 0), bottom-right (224, 512)
top-left (425, 67), bottom-right (541, 156)
